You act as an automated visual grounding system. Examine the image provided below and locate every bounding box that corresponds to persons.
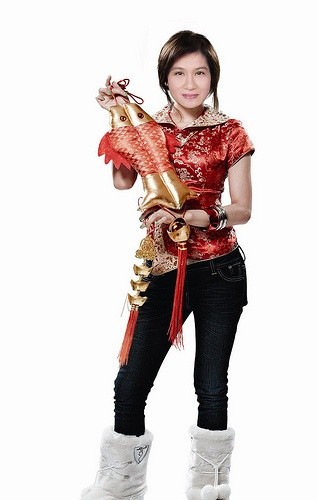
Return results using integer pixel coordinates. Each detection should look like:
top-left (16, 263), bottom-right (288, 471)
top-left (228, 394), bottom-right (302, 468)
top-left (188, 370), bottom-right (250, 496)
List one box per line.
top-left (79, 30), bottom-right (256, 500)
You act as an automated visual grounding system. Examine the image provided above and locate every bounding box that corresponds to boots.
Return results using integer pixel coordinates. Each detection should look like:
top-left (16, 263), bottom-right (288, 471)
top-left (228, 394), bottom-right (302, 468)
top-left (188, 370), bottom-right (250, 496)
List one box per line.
top-left (185, 425), bottom-right (235, 500)
top-left (80, 425), bottom-right (154, 500)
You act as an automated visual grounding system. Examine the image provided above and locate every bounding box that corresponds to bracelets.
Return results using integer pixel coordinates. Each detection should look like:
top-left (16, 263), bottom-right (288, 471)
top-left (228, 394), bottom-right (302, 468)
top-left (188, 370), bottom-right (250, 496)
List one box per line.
top-left (201, 204), bottom-right (228, 233)
